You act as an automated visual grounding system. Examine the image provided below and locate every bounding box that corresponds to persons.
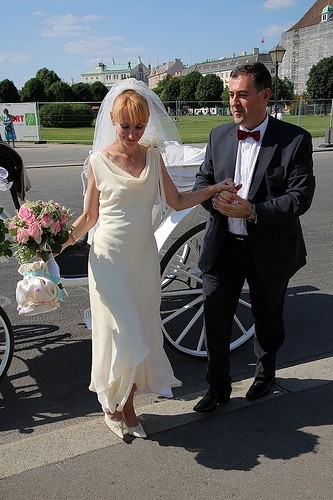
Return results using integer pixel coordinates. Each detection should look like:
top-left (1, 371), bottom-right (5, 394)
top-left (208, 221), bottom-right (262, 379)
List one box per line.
top-left (34, 78), bottom-right (238, 440)
top-left (191, 61), bottom-right (316, 412)
top-left (1, 108), bottom-right (17, 148)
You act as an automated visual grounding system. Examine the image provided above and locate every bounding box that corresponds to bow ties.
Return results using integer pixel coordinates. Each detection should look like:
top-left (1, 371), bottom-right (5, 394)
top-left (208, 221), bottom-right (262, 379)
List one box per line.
top-left (237, 130), bottom-right (260, 143)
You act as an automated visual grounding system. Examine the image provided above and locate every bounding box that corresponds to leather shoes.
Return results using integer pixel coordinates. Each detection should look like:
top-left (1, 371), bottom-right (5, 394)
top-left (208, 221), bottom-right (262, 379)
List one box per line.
top-left (193, 388), bottom-right (230, 411)
top-left (246, 379), bottom-right (275, 398)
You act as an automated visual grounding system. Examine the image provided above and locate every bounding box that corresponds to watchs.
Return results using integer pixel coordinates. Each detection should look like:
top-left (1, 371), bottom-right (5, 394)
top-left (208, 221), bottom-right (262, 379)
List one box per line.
top-left (246, 203), bottom-right (256, 222)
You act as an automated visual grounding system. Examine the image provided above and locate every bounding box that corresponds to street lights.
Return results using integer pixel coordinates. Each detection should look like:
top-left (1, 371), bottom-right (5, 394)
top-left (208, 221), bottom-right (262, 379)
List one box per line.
top-left (268, 44), bottom-right (286, 120)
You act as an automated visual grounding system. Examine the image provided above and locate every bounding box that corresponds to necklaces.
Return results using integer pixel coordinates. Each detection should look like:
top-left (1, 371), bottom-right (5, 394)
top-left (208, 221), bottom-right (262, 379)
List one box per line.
top-left (115, 142), bottom-right (142, 177)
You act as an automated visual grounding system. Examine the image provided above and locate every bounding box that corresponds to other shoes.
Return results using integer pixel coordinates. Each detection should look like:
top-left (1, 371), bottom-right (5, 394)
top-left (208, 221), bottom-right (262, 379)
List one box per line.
top-left (123, 421), bottom-right (147, 437)
top-left (105, 412), bottom-right (123, 439)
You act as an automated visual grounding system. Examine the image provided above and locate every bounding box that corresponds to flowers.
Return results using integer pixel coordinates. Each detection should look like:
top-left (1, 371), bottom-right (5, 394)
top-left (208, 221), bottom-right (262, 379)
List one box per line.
top-left (8, 198), bottom-right (75, 263)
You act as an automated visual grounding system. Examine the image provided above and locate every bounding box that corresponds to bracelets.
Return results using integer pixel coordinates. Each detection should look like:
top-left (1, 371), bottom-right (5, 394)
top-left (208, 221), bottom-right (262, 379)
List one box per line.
top-left (70, 233), bottom-right (75, 246)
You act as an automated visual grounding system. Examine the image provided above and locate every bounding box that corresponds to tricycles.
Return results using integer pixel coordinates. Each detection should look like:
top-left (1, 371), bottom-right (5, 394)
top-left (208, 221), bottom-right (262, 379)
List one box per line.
top-left (0, 156), bottom-right (256, 383)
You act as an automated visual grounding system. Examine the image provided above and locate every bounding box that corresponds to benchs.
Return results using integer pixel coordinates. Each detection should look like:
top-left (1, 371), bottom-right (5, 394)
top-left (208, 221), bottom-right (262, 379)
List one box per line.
top-left (151, 145), bottom-right (210, 239)
